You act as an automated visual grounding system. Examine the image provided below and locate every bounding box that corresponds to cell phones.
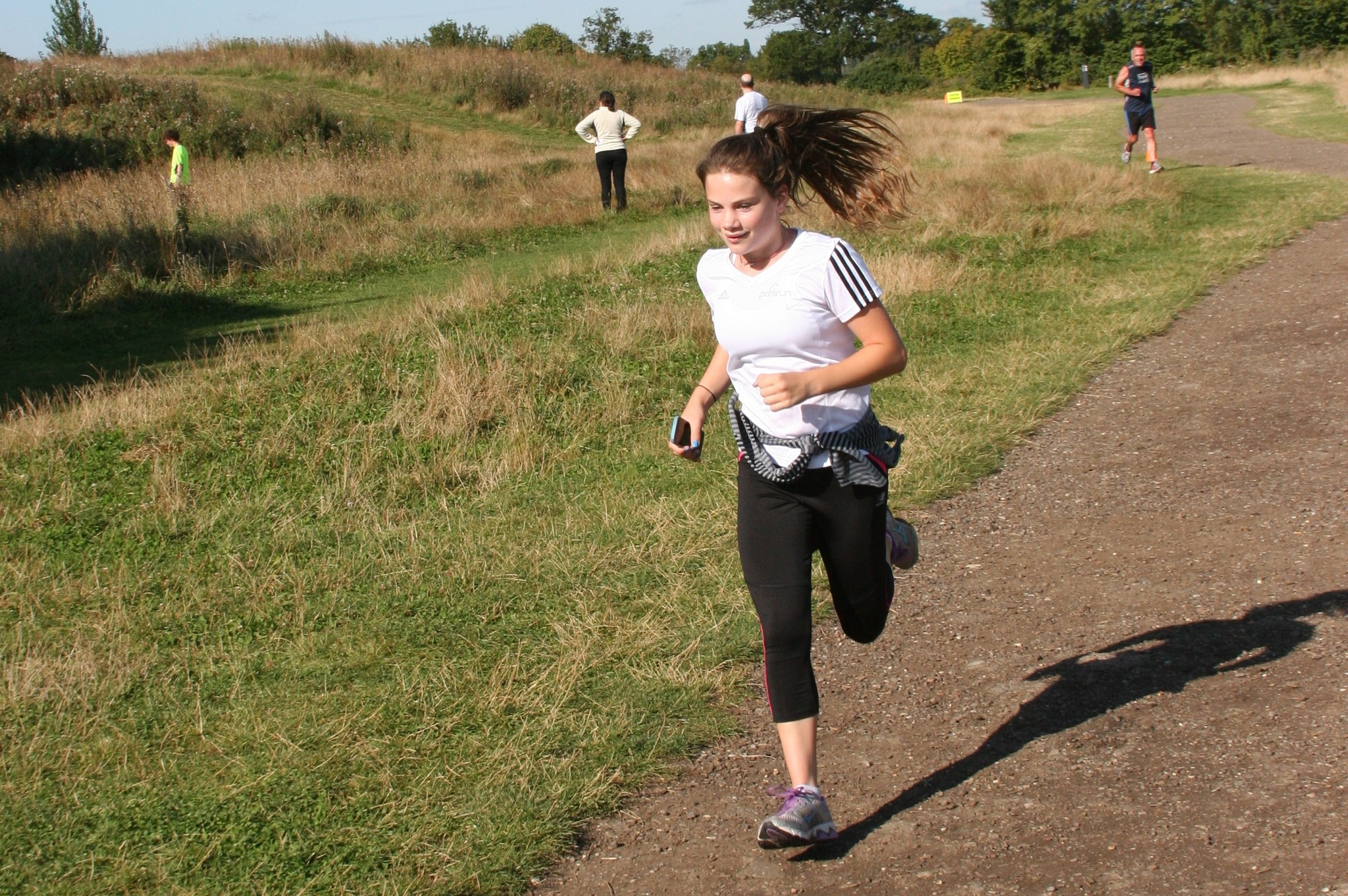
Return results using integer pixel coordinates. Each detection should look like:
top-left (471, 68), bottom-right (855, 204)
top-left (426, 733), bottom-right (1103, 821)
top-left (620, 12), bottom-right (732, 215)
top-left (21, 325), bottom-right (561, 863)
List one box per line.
top-left (670, 416), bottom-right (704, 458)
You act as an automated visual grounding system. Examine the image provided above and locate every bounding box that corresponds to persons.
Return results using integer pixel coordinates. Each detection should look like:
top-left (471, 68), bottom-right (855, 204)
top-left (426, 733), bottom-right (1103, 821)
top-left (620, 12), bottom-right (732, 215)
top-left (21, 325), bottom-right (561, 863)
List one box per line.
top-left (575, 91), bottom-right (641, 214)
top-left (734, 74), bottom-right (767, 135)
top-left (668, 104), bottom-right (919, 850)
top-left (163, 129), bottom-right (191, 232)
top-left (1114, 47), bottom-right (1164, 174)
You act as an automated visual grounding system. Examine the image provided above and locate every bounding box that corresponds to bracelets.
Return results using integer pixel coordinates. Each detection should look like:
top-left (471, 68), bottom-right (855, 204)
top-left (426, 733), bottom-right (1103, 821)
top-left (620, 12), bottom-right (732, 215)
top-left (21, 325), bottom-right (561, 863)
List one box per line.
top-left (697, 385), bottom-right (717, 402)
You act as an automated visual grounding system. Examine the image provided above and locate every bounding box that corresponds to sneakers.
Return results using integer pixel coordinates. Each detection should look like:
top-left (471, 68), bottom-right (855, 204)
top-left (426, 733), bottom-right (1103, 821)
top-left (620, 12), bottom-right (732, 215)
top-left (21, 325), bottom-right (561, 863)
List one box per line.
top-left (1150, 162), bottom-right (1163, 173)
top-left (884, 505), bottom-right (918, 570)
top-left (1121, 144), bottom-right (1131, 163)
top-left (757, 784), bottom-right (837, 849)
